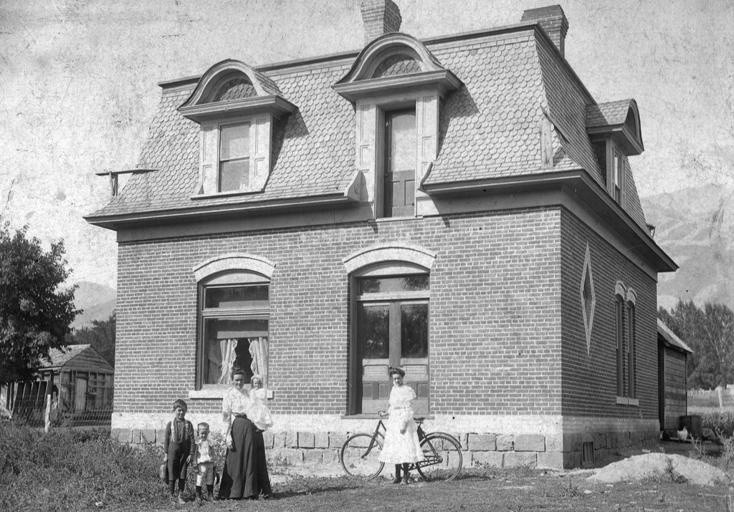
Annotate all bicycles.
[342,409,463,483]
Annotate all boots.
[392,463,408,485]
[167,479,185,505]
[195,485,203,506]
[206,483,217,505]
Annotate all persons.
[248,374,273,432]
[161,398,194,504]
[376,365,425,486]
[191,421,218,505]
[219,367,271,500]
